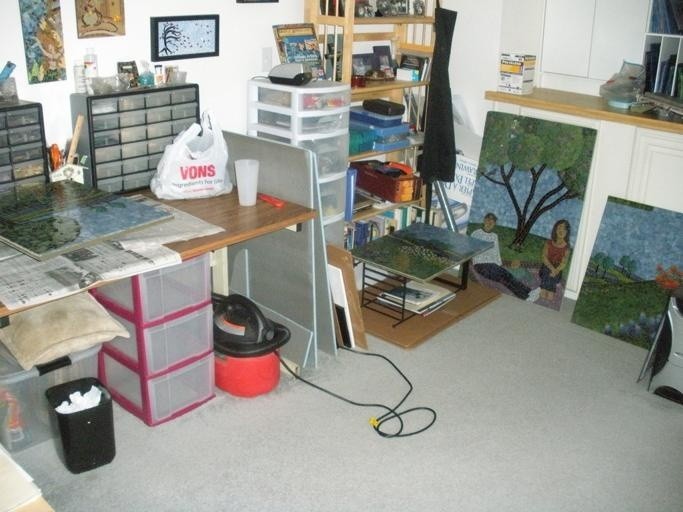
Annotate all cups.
[234,159,258,206]
[72,65,86,94]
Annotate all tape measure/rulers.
[67,115,84,164]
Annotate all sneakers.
[525,287,541,303]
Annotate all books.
[646,44,683,99]
[345,205,425,246]
[379,281,455,317]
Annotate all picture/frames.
[150,14,219,62]
[273,23,329,82]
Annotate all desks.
[345,223,494,328]
[0,178,318,324]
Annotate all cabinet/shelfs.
[640,0,682,110]
[303,0,436,225]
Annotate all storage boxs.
[246,74,351,250]
[69,82,200,196]
[0,341,103,454]
[87,253,218,427]
[0,99,48,197]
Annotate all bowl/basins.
[107,72,129,92]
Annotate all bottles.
[154,65,162,84]
[83,48,99,95]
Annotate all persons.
[470,213,542,303]
[539,221,571,301]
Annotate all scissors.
[50,144,59,172]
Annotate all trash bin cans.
[45,377,115,474]
